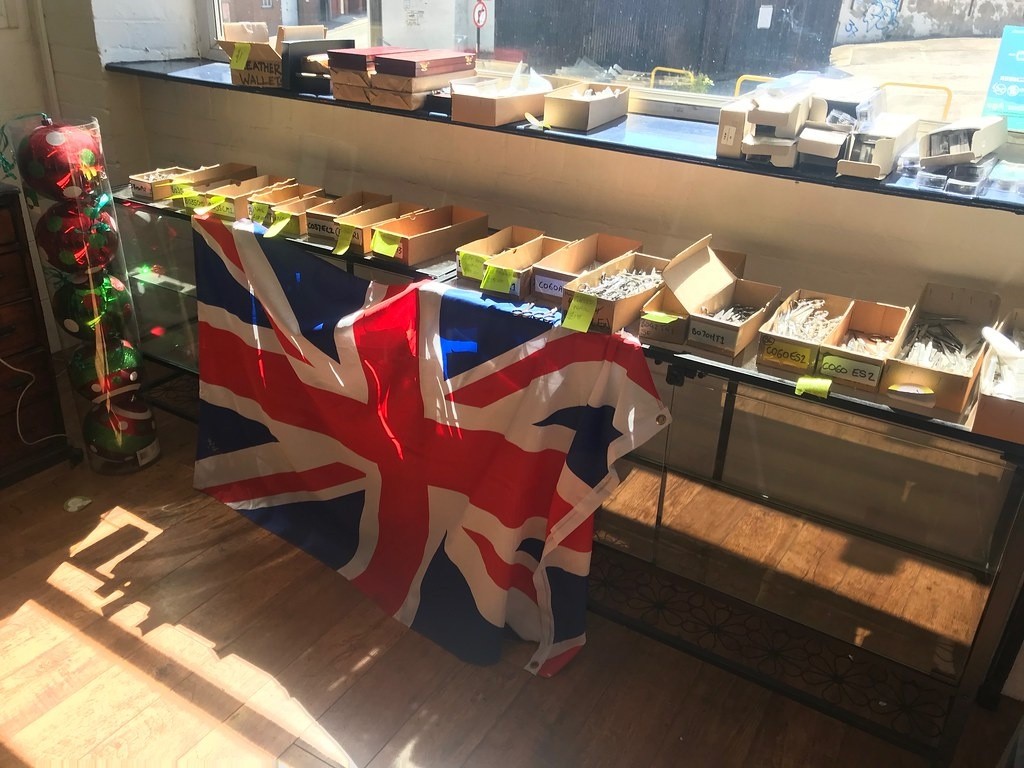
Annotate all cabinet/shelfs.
[111,187,1024,768]
[0,191,69,493]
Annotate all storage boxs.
[129,23,1024,443]
